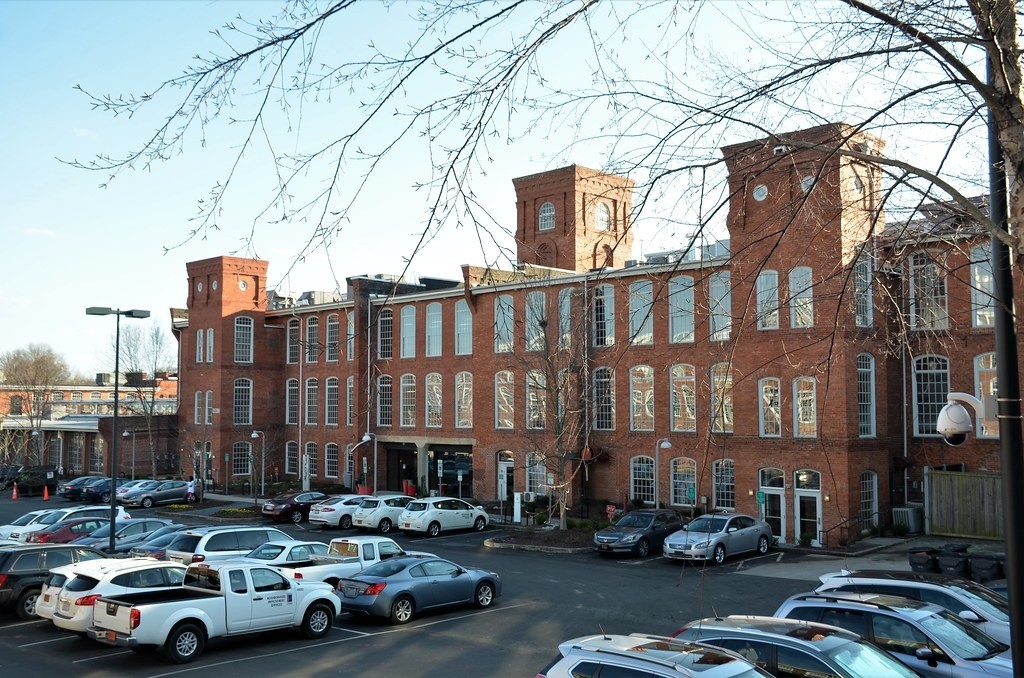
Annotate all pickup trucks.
[88,562,341,665]
[269,537,442,590]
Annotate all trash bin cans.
[970,556,1004,583]
[910,542,971,577]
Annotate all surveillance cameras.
[936,402,973,446]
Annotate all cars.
[58,475,204,509]
[339,556,503,626]
[352,494,418,533]
[70,519,184,559]
[35,555,158,639]
[262,491,332,523]
[398,497,490,539]
[308,495,373,529]
[662,513,773,563]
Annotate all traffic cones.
[11,482,19,499]
[42,486,49,500]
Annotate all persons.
[187,476,195,503]
[358,474,364,487]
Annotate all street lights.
[251,429,265,497]
[361,432,378,495]
[655,437,673,510]
[32,430,44,467]
[123,431,136,480]
[85,305,151,552]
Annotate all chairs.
[300,548,307,557]
[716,522,724,532]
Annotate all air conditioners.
[524,492,537,502]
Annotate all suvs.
[0,544,113,621]
[783,571,1014,678]
[165,526,294,567]
[593,509,690,558]
[536,633,759,678]
[673,614,924,678]
[0,505,132,549]
